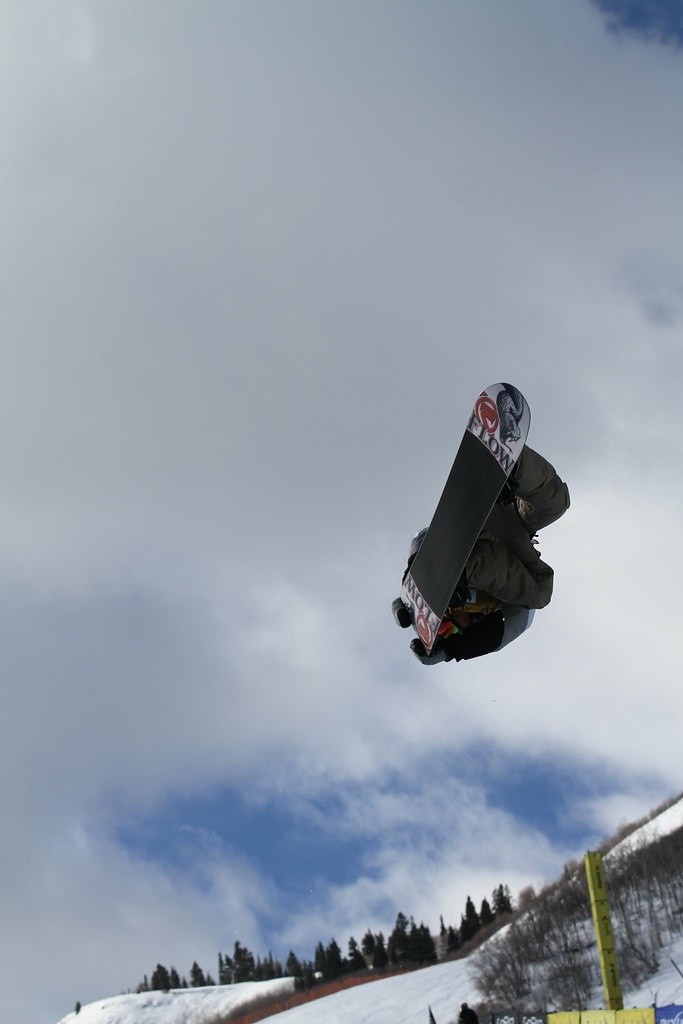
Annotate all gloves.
[410,639,446,665]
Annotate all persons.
[458,1003,480,1024]
[392,443,571,666]
[491,1012,496,1024]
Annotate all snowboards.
[401,382,531,658]
